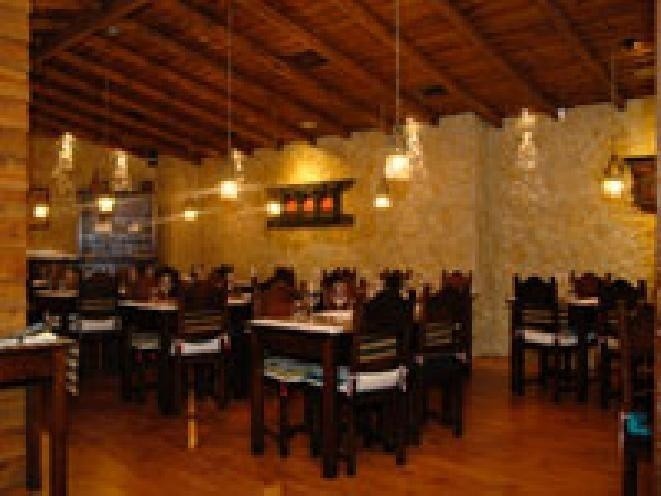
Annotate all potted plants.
[630,156,660,215]
[24,187,51,231]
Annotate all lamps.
[598,278,650,405]
[114,265,161,403]
[68,273,125,371]
[339,288,416,477]
[566,267,612,298]
[319,266,367,310]
[169,279,230,411]
[377,266,416,294]
[250,274,306,316]
[417,270,473,439]
[617,299,654,495]
[26,276,64,332]
[506,272,558,396]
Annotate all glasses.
[218,4,240,200]
[373,101,392,208]
[601,53,625,202]
[32,188,51,223]
[96,179,117,216]
[385,3,411,180]
[263,188,281,217]
[183,175,201,222]
